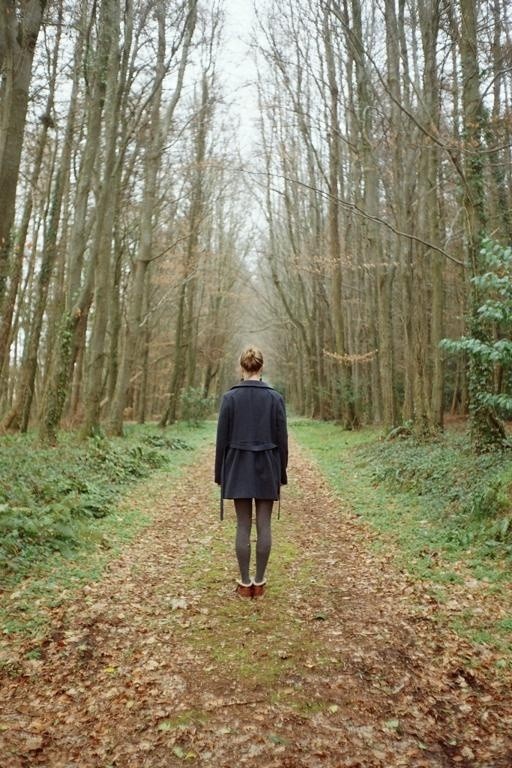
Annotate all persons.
[215,348,289,600]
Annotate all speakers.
[253,577,267,601]
[235,578,253,602]
